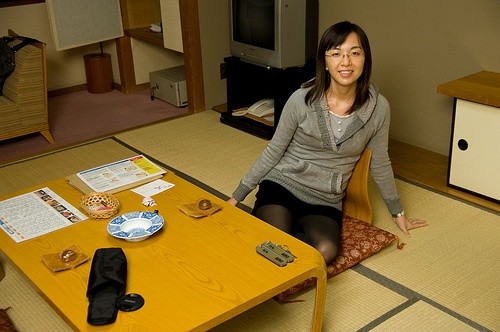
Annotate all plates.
[107,211,164,242]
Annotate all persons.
[226,20,430,264]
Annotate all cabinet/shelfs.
[119,0,185,54]
[219,57,312,142]
[436,69,499,205]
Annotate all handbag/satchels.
[0,36,34,96]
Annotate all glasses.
[324,50,365,58]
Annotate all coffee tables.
[0,155,328,332]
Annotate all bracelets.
[391,210,406,218]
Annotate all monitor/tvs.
[230,0,318,70]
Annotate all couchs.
[0,29,54,145]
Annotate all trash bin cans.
[83,53,114,94]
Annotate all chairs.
[344,149,373,224]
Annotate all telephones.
[248,99,274,117]
